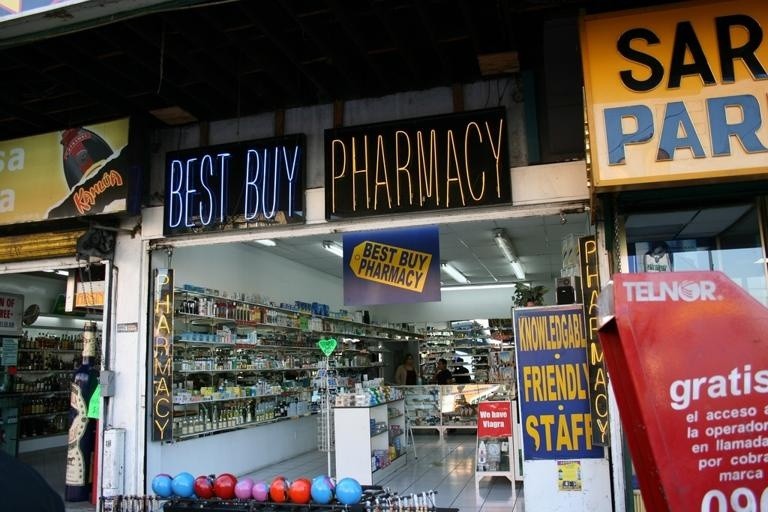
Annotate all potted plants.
[512,281,547,306]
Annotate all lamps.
[493,230,520,263]
[320,238,343,258]
[440,261,471,286]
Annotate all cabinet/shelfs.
[332,397,408,488]
[472,397,520,506]
[22,328,103,446]
[170,295,425,437]
[392,319,516,444]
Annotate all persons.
[1,447,65,512]
[451,358,471,383]
[395,354,418,385]
[433,359,451,383]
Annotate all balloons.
[152,473,173,498]
[171,472,195,497]
[310,476,334,504]
[288,477,312,504]
[194,475,213,499]
[214,474,236,499]
[270,476,290,503]
[233,478,254,499]
[334,478,363,506]
[251,480,269,502]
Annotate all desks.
[0,392,22,461]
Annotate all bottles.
[15,320,98,503]
[172,395,319,438]
[178,284,380,338]
[58,126,115,192]
[478,437,488,467]
